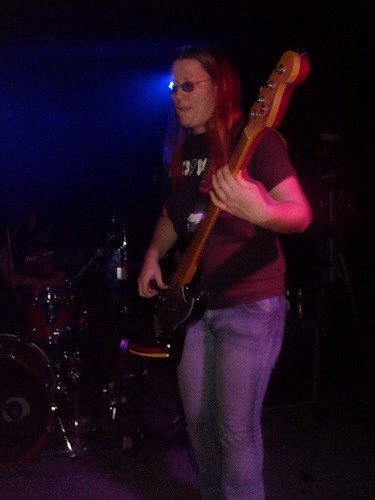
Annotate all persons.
[137,44,312,500]
[0,207,66,333]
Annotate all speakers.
[261,321,321,412]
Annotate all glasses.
[168,77,212,94]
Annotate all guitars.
[144,50,310,349]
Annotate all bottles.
[284,288,305,321]
[112,229,130,284]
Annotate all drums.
[13,270,76,340]
[0,332,56,468]
